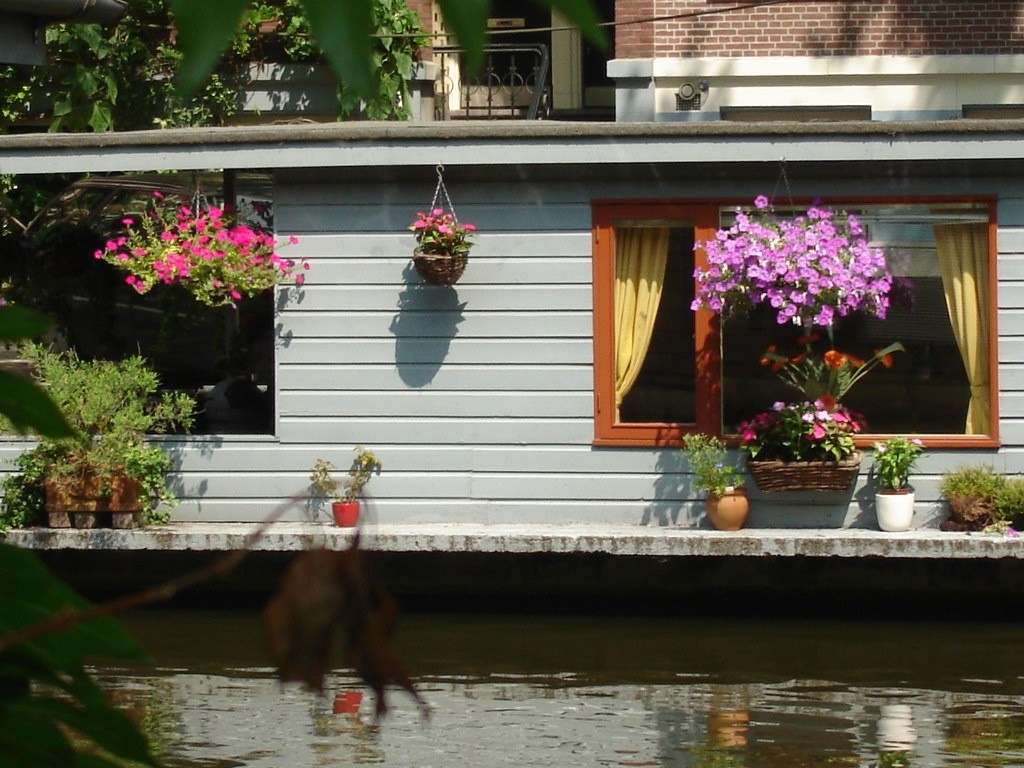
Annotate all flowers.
[92,180,312,312]
[736,393,866,462]
[686,190,894,330]
[406,207,477,253]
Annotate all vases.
[413,248,468,286]
[748,454,861,494]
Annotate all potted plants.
[687,709,749,768]
[16,337,204,530]
[865,435,928,532]
[309,444,381,529]
[680,433,749,531]
[873,703,916,768]
[309,689,385,766]
